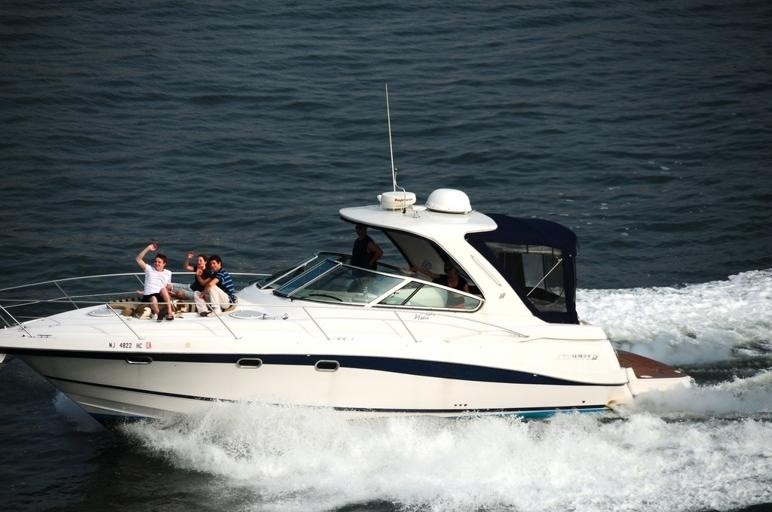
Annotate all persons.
[352,223,383,291]
[411,260,469,307]
[194,255,237,317]
[176,251,211,299]
[136,242,174,320]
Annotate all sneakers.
[157,312,174,321]
[199,310,222,317]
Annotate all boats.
[0,82,700,448]
